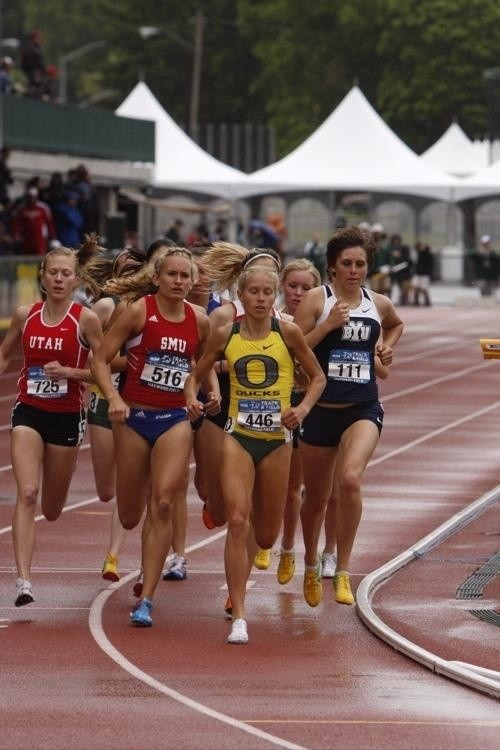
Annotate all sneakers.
[14,578,34,607]
[102,504,355,644]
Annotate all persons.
[0,147,104,213]
[1,215,413,646]
[413,241,436,307]
[0,24,58,106]
[467,235,500,299]
